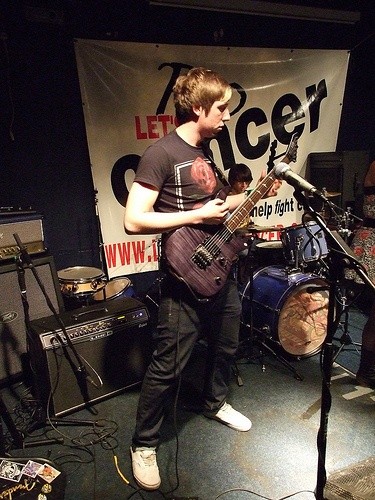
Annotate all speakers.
[27,295,154,418]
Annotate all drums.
[293,221,330,262]
[241,265,344,358]
[253,237,288,265]
[56,265,105,310]
[96,276,136,303]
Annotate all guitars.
[160,131,299,302]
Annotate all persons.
[123,67,282,490]
[225,163,267,256]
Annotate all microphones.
[77,368,94,386]
[275,162,329,202]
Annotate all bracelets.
[245,188,254,198]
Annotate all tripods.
[232,233,303,380]
[332,289,362,362]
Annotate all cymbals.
[233,221,286,232]
[309,188,342,199]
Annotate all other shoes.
[130,444,161,489]
[214,402,252,432]
